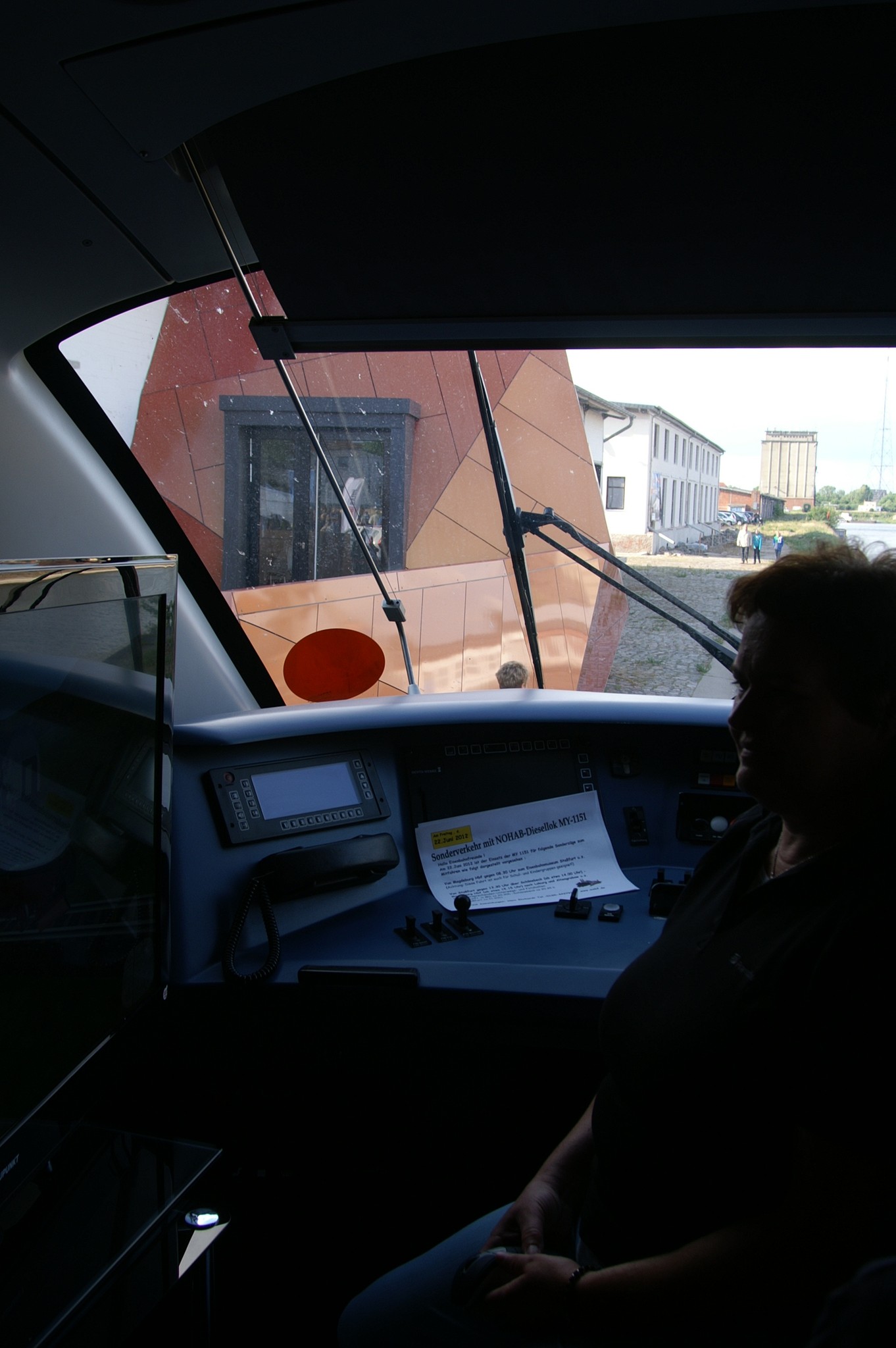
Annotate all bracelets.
[568,1265,594,1288]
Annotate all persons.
[736,524,752,564]
[773,531,784,561]
[342,540,896,1348]
[752,530,762,564]
[753,511,763,526]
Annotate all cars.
[718,510,759,526]
